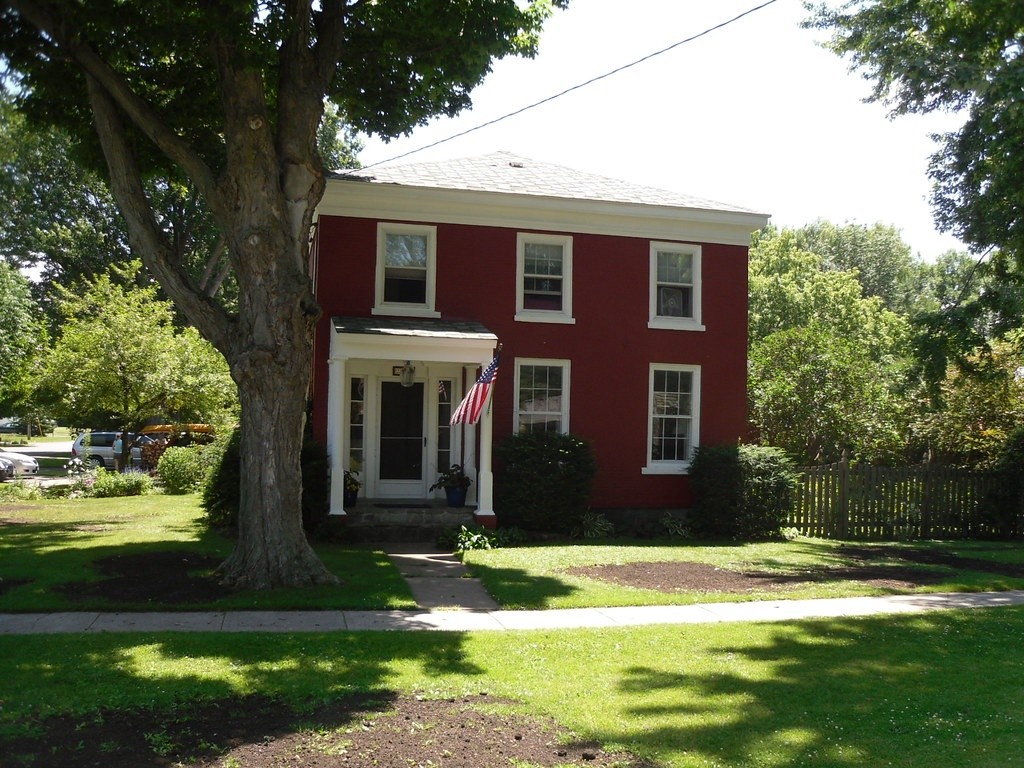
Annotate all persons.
[112,432,123,469]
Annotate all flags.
[450,355,499,426]
[439,382,445,394]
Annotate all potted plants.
[428,463,474,507]
[343,469,364,508]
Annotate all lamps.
[400,360,416,386]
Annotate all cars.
[0,448,39,483]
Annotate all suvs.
[71,424,214,471]
